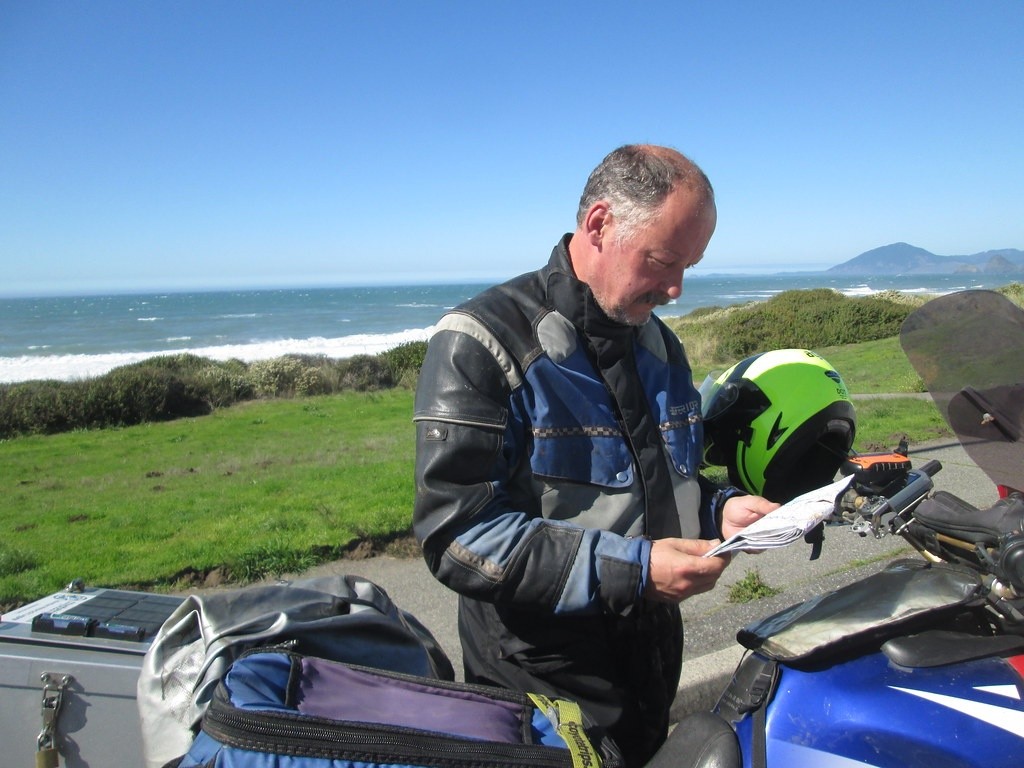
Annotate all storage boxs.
[1,585,202,768]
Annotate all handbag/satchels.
[177,646,622,768]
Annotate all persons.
[412,144,780,768]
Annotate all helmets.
[699,348,855,502]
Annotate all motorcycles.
[137,288,1024,768]
